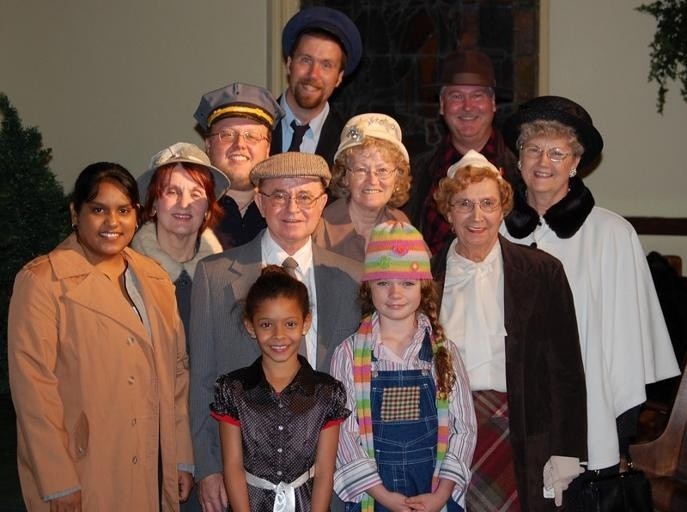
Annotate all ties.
[287,119,311,153]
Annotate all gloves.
[542,455,581,507]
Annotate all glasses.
[517,141,578,163]
[258,191,327,208]
[447,199,503,213]
[343,164,401,179]
[204,130,272,144]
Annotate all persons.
[7,162,196,512]
[328,219,478,512]
[269,6,363,205]
[402,49,525,255]
[208,265,352,512]
[432,148,585,511]
[192,82,286,250]
[313,112,412,263]
[127,141,233,358]
[189,152,363,512]
[502,96,682,489]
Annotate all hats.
[446,148,503,180]
[142,141,231,203]
[500,94,605,181]
[360,217,435,283]
[431,48,497,88]
[333,113,410,166]
[282,5,364,77]
[249,151,332,190]
[193,81,287,140]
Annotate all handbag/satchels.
[566,466,654,512]
